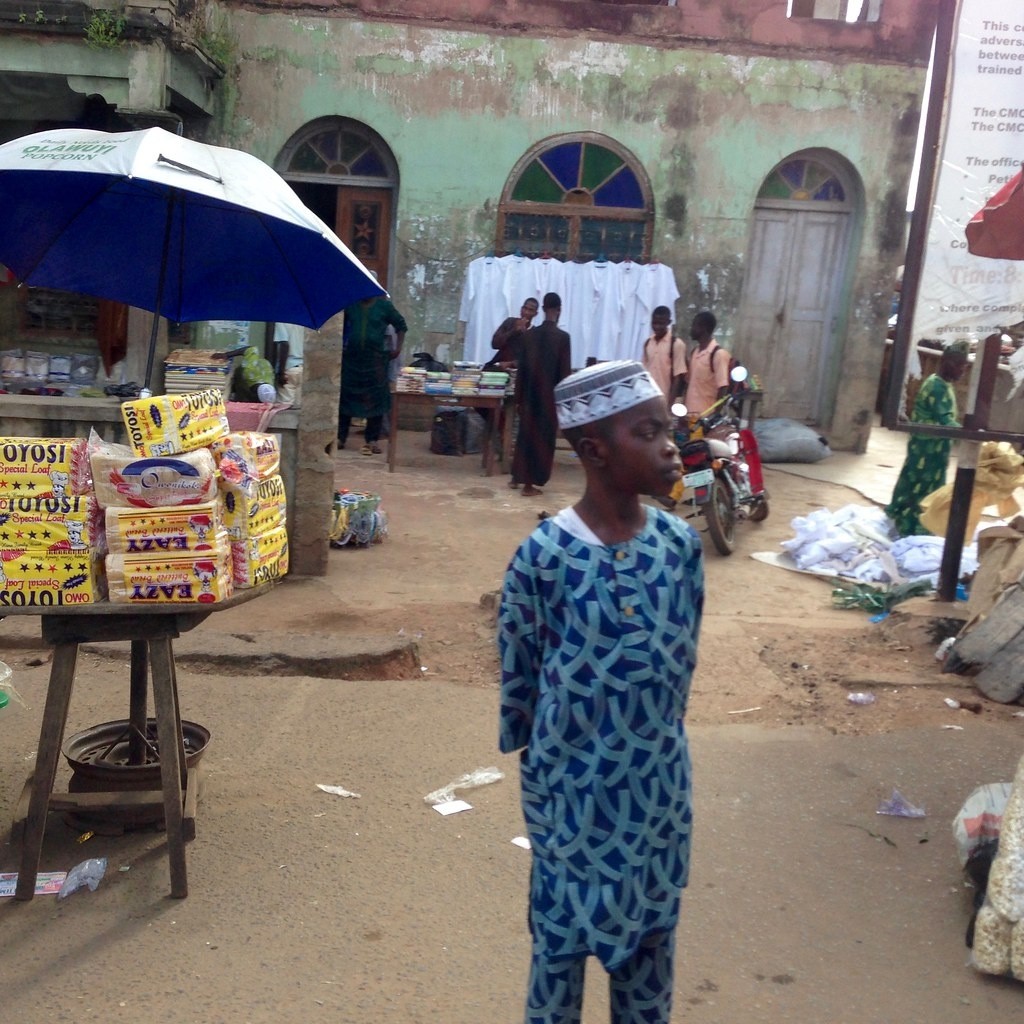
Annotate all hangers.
[624,253,630,271]
[648,252,659,270]
[572,253,582,262]
[595,251,608,268]
[514,247,522,257]
[539,248,550,259]
[484,245,494,257]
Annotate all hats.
[555,360,666,430]
[943,340,971,355]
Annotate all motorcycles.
[670,366,773,554]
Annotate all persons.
[886,339,971,538]
[509,292,574,497]
[502,351,709,1020]
[643,306,687,416]
[330,269,409,456]
[474,297,540,462]
[651,311,732,511]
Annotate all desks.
[1,579,277,904]
[735,384,765,429]
[388,381,515,477]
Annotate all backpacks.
[710,345,747,409]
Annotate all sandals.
[360,446,371,455]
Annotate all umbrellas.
[0,125,393,446]
[963,159,1024,264]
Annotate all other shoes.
[369,442,382,454]
